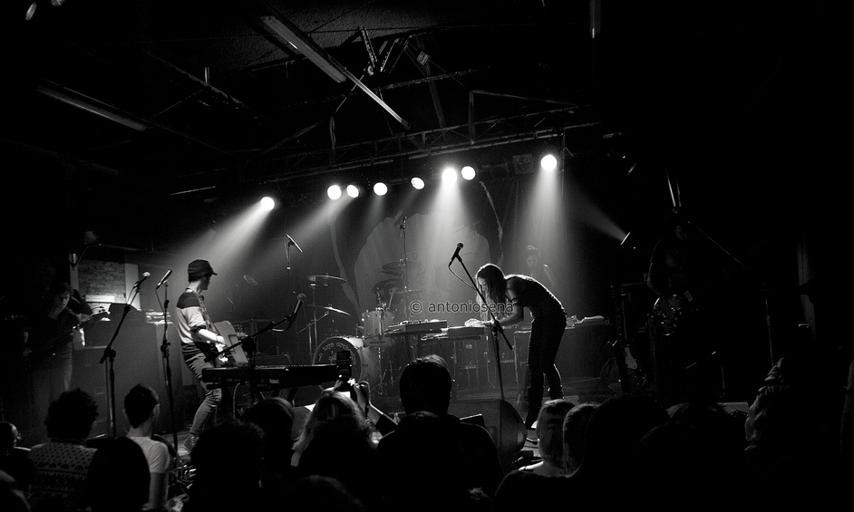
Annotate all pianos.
[201,364,340,383]
[383,319,448,340]
[426,325,488,341]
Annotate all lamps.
[259,190,281,212]
[461,160,479,182]
[346,182,364,198]
[326,182,343,201]
[538,143,560,172]
[411,171,427,191]
[373,180,389,196]
[440,160,459,181]
[618,231,640,250]
[16,2,37,20]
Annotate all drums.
[312,336,380,394]
[358,309,400,349]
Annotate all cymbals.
[321,304,349,319]
[309,274,345,288]
[393,287,421,301]
[381,259,417,278]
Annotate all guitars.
[602,288,646,391]
[202,322,232,367]
[52,307,110,352]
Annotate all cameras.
[333,348,367,403]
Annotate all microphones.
[133,270,151,288]
[282,231,304,256]
[155,266,174,290]
[284,292,307,331]
[448,242,464,267]
[400,215,408,231]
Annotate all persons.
[24,283,85,443]
[176,259,226,454]
[86,436,151,512]
[292,379,397,512]
[554,403,601,465]
[24,389,98,512]
[377,354,503,511]
[124,385,170,512]
[520,245,558,294]
[238,397,294,470]
[474,264,567,428]
[492,401,574,510]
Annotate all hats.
[187,260,218,282]
